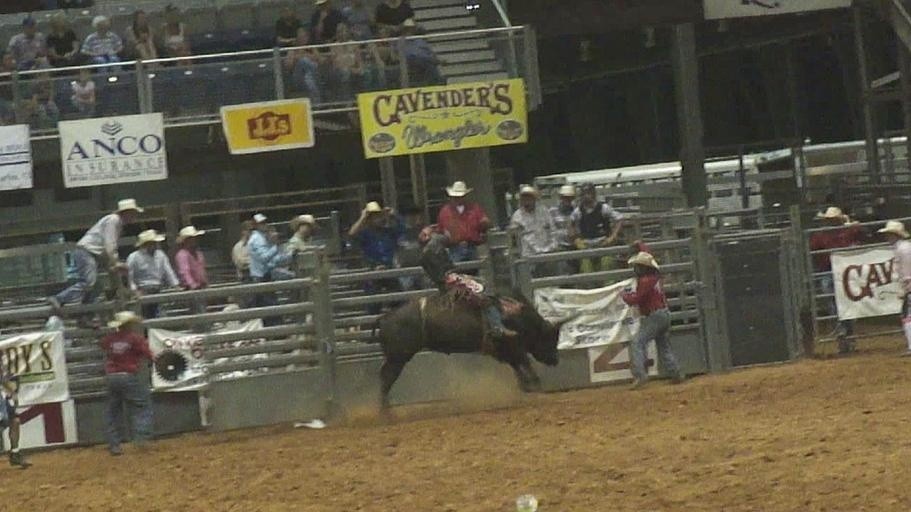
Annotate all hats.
[418,223,450,244]
[176,226,205,243]
[876,221,911,239]
[252,214,268,224]
[815,207,845,225]
[557,186,576,197]
[112,198,145,213]
[135,229,166,247]
[366,201,383,212]
[289,215,316,231]
[107,311,142,328]
[627,251,659,271]
[445,182,474,197]
[514,187,543,201]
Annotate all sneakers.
[9,452,33,467]
[629,374,648,390]
[493,326,518,336]
[672,374,685,383]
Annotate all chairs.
[0,0,439,130]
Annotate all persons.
[173,225,210,333]
[126,229,187,320]
[0,383,24,466]
[811,206,861,353]
[876,220,911,357]
[0,0,194,130]
[508,183,624,289]
[274,1,416,105]
[98,311,158,457]
[435,181,489,277]
[47,198,143,330]
[232,213,320,338]
[416,223,519,336]
[348,201,408,338]
[621,239,685,390]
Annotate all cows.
[371,284,585,421]
